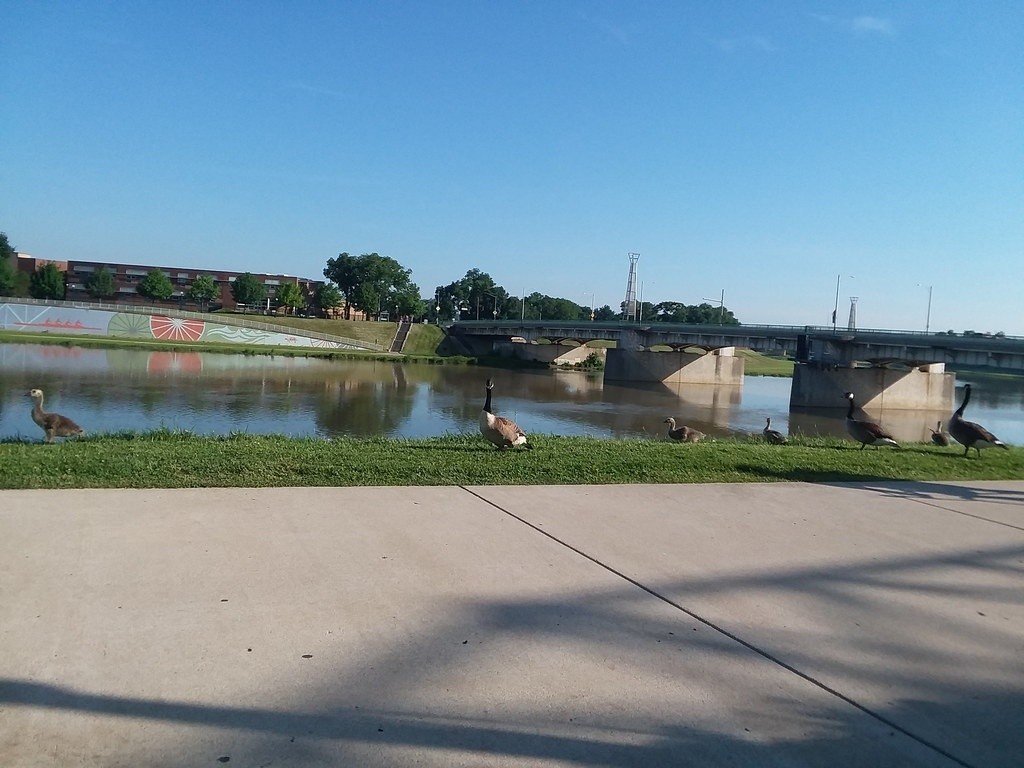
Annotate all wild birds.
[948,384,1010,457]
[928,421,950,447]
[664,417,707,443]
[840,392,902,450]
[479,379,533,452]
[762,418,788,445]
[24,389,84,442]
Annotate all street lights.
[352,302,357,321]
[71,284,75,301]
[275,297,279,317]
[179,291,183,309]
